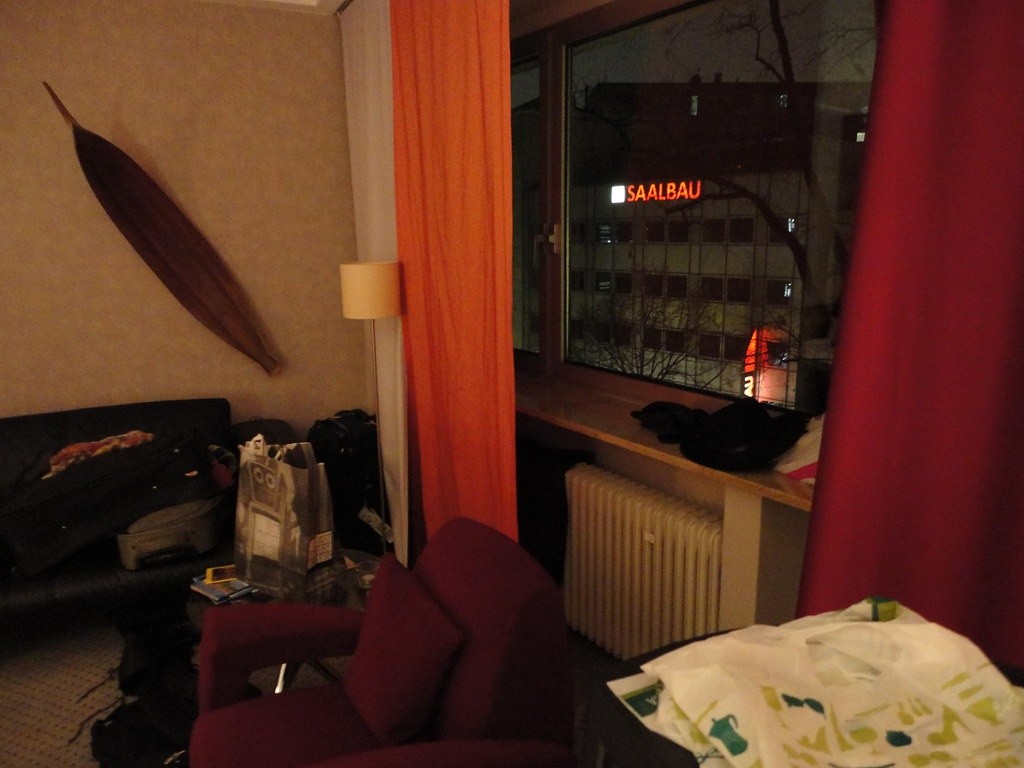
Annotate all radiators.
[562,461,723,662]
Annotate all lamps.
[340,260,402,555]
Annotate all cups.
[356,561,378,589]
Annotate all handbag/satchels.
[234,433,335,577]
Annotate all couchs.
[0,398,300,652]
[191,518,581,768]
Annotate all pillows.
[343,552,464,753]
